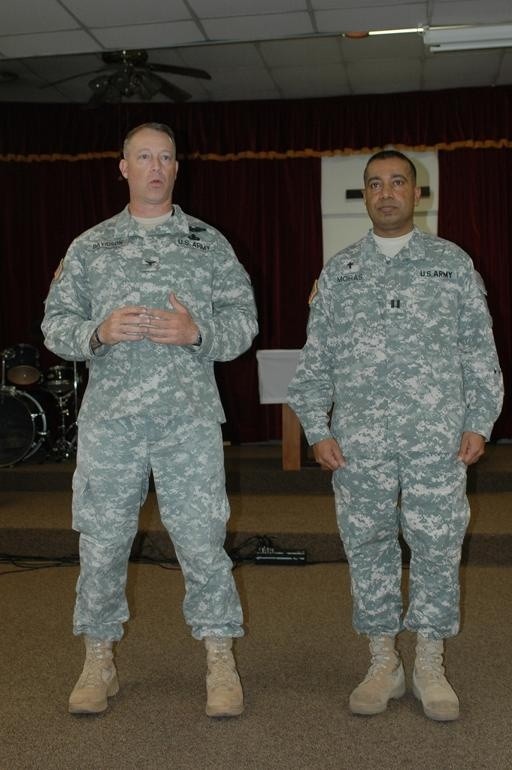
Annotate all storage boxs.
[256,348,305,404]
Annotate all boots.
[412,632,460,722]
[349,636,406,715]
[204,635,245,719]
[68,634,120,715]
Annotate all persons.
[41,123,261,716]
[287,149,504,722]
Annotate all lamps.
[89,49,152,102]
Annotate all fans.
[37,49,211,103]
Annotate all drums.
[0,344,79,468]
